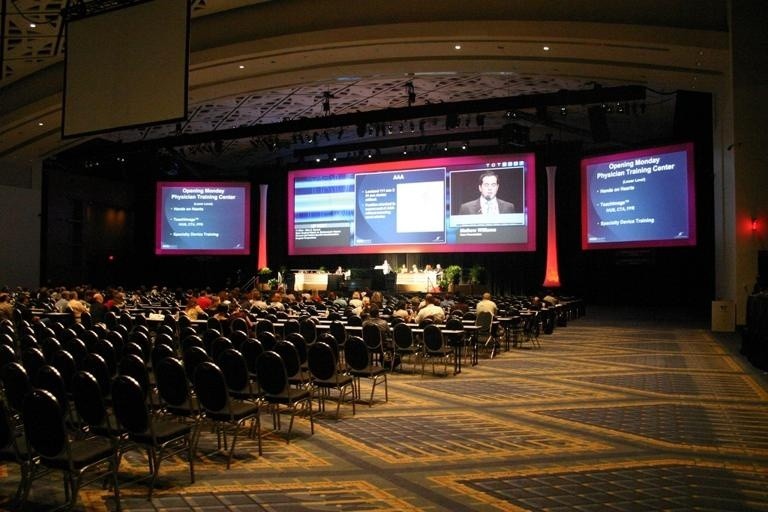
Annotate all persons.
[475,292,499,329]
[370,290,385,309]
[329,291,347,308]
[364,286,372,296]
[523,296,540,308]
[416,294,446,323]
[392,301,413,322]
[212,303,231,327]
[181,300,209,327]
[542,290,557,333]
[459,170,514,214]
[101,279,320,311]
[440,293,458,308]
[1,284,106,324]
[362,303,392,350]
[229,303,259,337]
[349,291,365,309]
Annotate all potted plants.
[437,278,448,291]
[269,278,278,290]
[257,267,271,284]
[469,265,486,296]
[445,265,461,294]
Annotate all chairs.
[215,349,277,439]
[390,323,423,377]
[286,334,312,389]
[111,375,196,500]
[1,363,28,433]
[29,366,76,429]
[153,360,215,454]
[276,341,319,420]
[183,288,317,347]
[316,333,356,402]
[53,350,75,396]
[183,346,213,407]
[22,389,122,512]
[445,321,467,370]
[115,357,167,421]
[71,372,148,469]
[489,295,587,352]
[346,337,389,407]
[22,348,46,379]
[329,322,352,365]
[420,326,452,378]
[1,345,17,368]
[74,354,118,416]
[469,311,499,360]
[0,397,41,507]
[0,285,180,359]
[253,350,314,444]
[362,294,483,320]
[309,343,356,422]
[239,340,266,372]
[194,363,263,470]
[361,323,392,371]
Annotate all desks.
[320,295,361,331]
